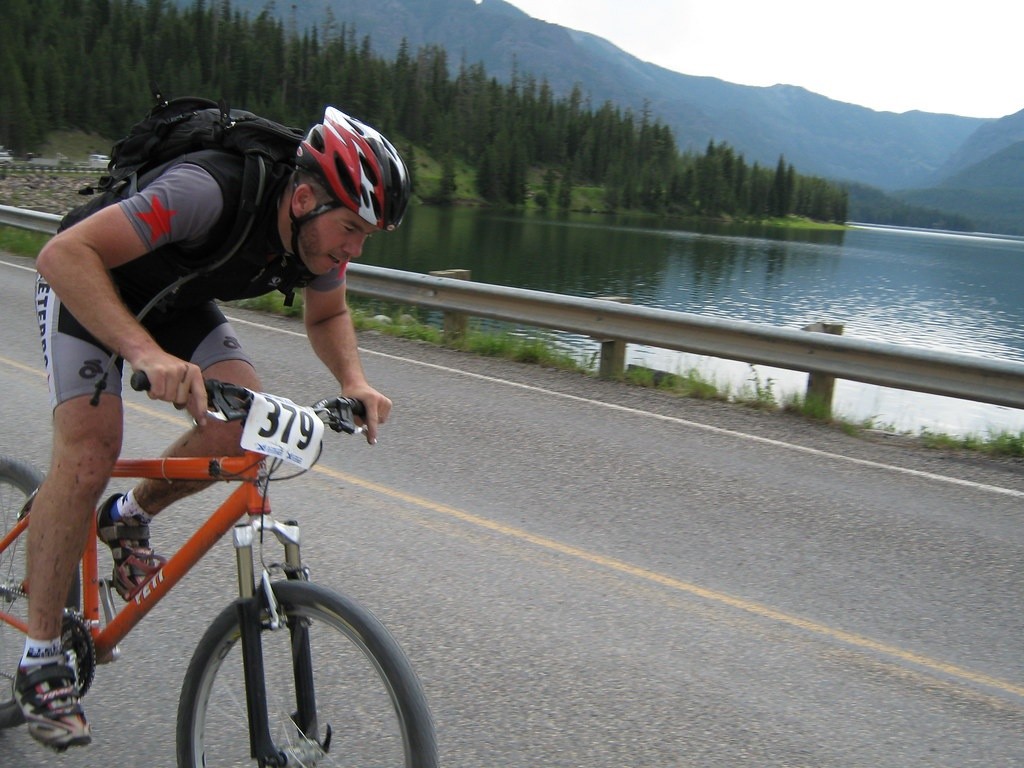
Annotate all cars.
[0,152,13,165]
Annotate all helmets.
[295,106,411,231]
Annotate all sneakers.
[96,492,166,601]
[12,654,92,749]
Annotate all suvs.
[88,154,110,164]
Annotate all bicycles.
[0,369,440,768]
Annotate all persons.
[11,105,409,750]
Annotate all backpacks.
[78,81,316,308]
